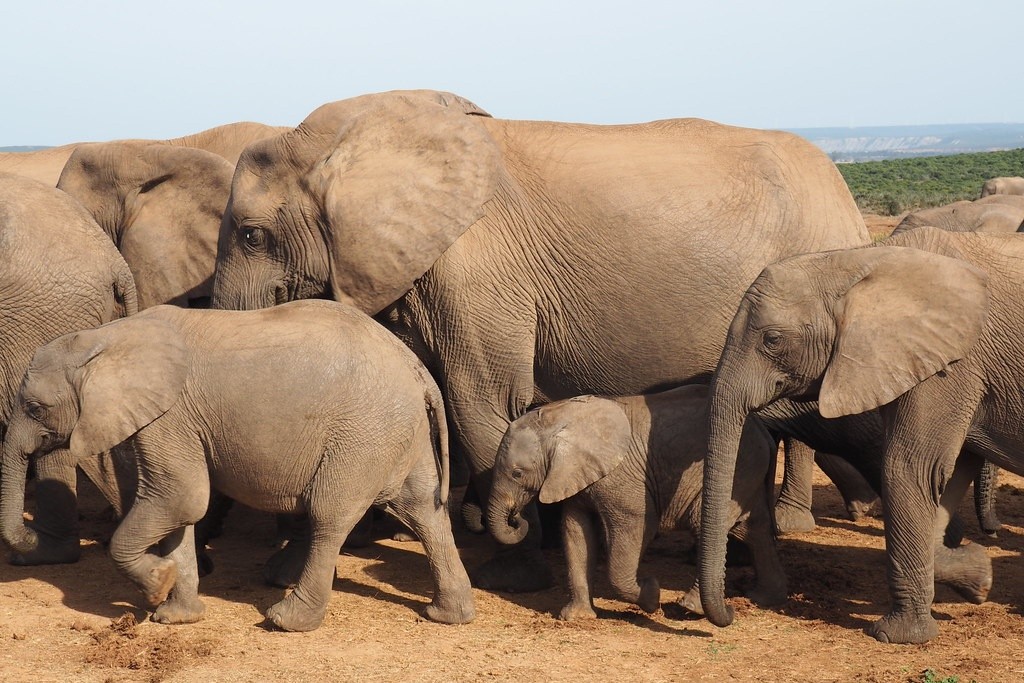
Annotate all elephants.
[1,89,1023,645]
